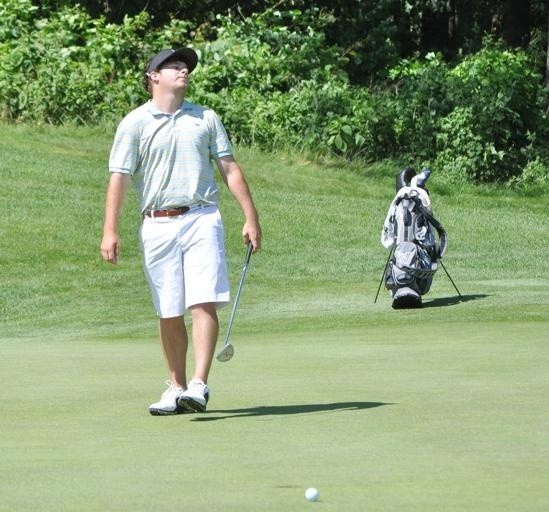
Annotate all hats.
[144,47,198,77]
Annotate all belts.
[145,202,209,219]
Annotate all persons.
[99,46,263,416]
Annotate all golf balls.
[305,488,319,500]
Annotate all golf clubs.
[216,241,252,362]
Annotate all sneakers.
[149,379,183,413]
[178,379,210,413]
